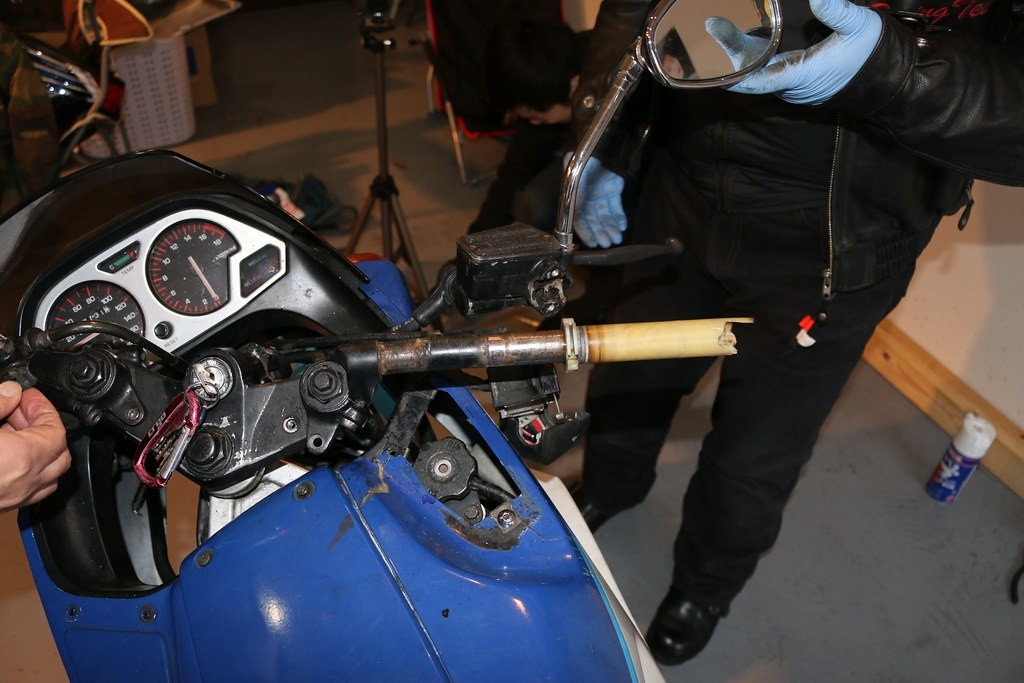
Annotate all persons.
[431,0,1024,666]
[0,379,72,510]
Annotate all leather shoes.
[569,478,625,533]
[646,583,721,665]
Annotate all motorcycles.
[0,2,782,683]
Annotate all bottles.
[11,54,60,200]
[925,412,997,503]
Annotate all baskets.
[78,36,196,157]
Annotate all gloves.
[719,0,882,106]
[565,152,629,249]
[705,16,770,71]
